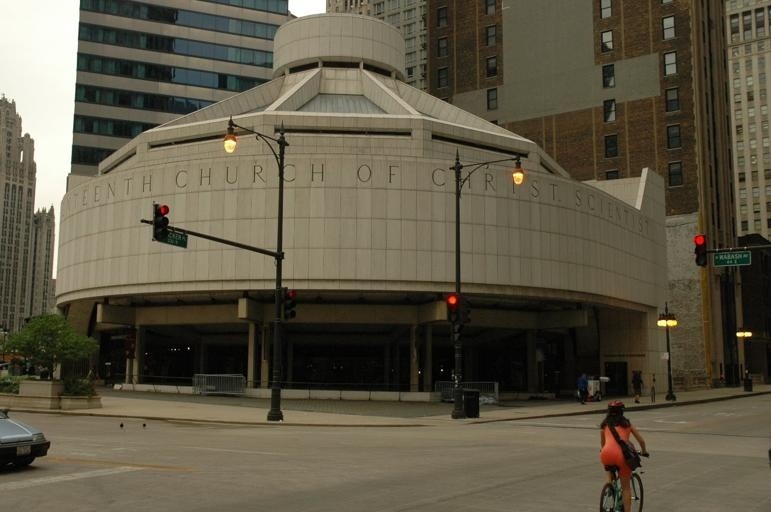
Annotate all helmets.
[607,400,625,408]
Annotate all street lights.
[656,302,680,401]
[0,329,9,361]
[449,147,531,422]
[222,114,286,422]
[733,326,755,394]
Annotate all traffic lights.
[443,291,462,329]
[150,203,171,243]
[691,235,709,271]
[280,285,297,324]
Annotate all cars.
[0,408,49,471]
[0,359,42,375]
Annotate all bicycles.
[590,448,651,512]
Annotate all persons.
[600,399,650,511]
[630,370,646,403]
[579,373,589,405]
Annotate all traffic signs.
[712,252,751,268]
[152,226,186,249]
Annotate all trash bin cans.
[463,389,480,419]
[744,379,753,392]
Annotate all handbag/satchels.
[623,446,641,471]
